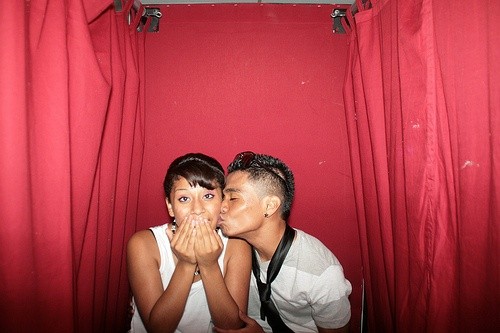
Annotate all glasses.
[227,150,290,195]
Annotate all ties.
[252,223,296,333]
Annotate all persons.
[126,153,252,333]
[217,151,353,333]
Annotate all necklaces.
[171,218,218,277]
